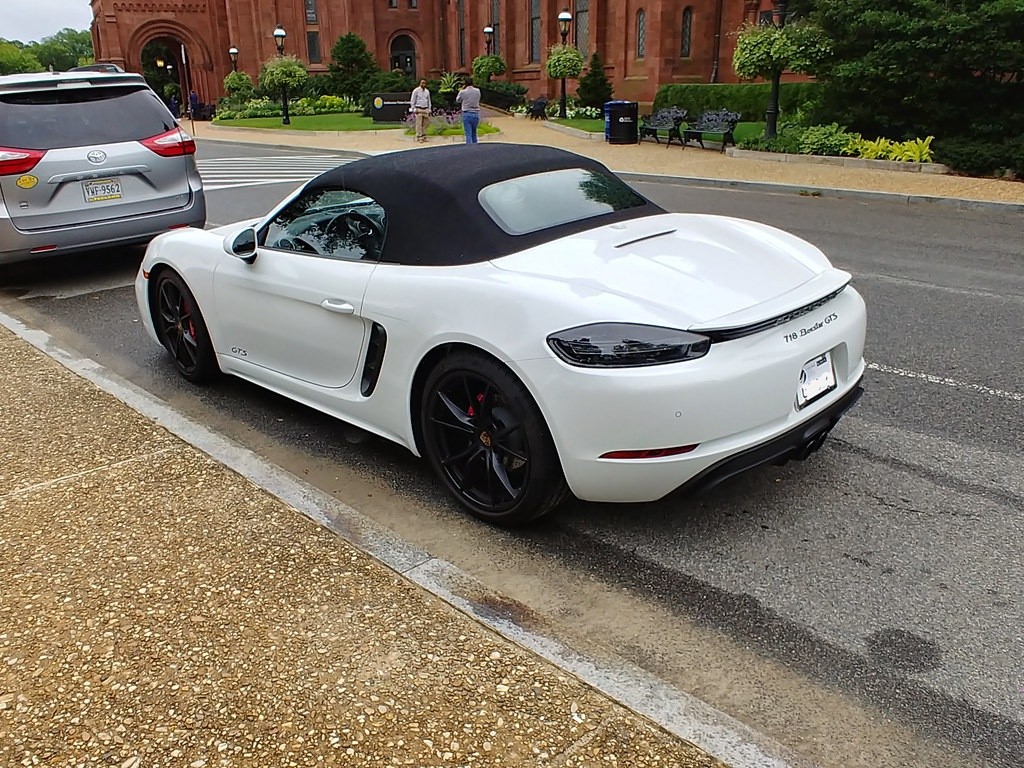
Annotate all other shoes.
[424,140,428,142]
[419,139,423,143]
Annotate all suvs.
[0,64,206,265]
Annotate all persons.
[170,95,179,118]
[411,79,431,143]
[187,96,191,120]
[456,78,480,143]
[190,90,198,120]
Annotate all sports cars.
[135,142,870,529]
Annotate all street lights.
[273,25,291,124]
[484,24,494,83]
[556,7,573,117]
[228,45,239,72]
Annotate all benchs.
[527,96,548,121]
[638,108,688,149]
[682,110,742,154]
[188,104,216,121]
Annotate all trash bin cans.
[190,102,205,120]
[604,99,638,145]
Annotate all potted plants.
[438,70,462,113]
